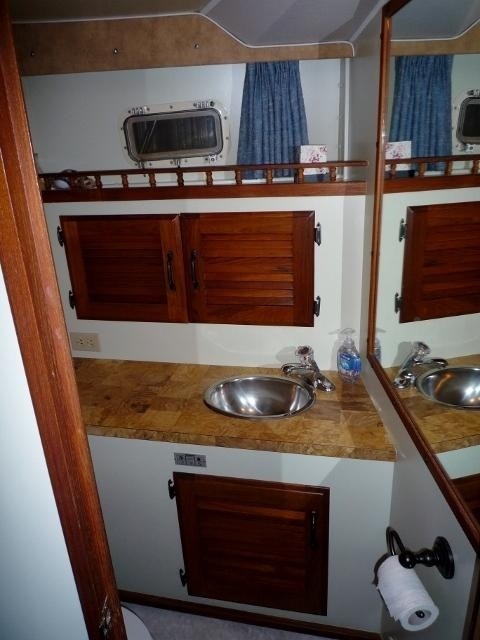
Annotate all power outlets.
[70,332,100,352]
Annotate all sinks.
[415,362,479,410]
[202,373,316,419]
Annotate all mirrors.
[367,0,480,556]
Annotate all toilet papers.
[374,554,440,631]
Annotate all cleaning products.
[336,326,361,383]
[373,326,387,362]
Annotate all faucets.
[392,342,450,390]
[281,346,335,392]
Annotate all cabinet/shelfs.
[168,473,330,616]
